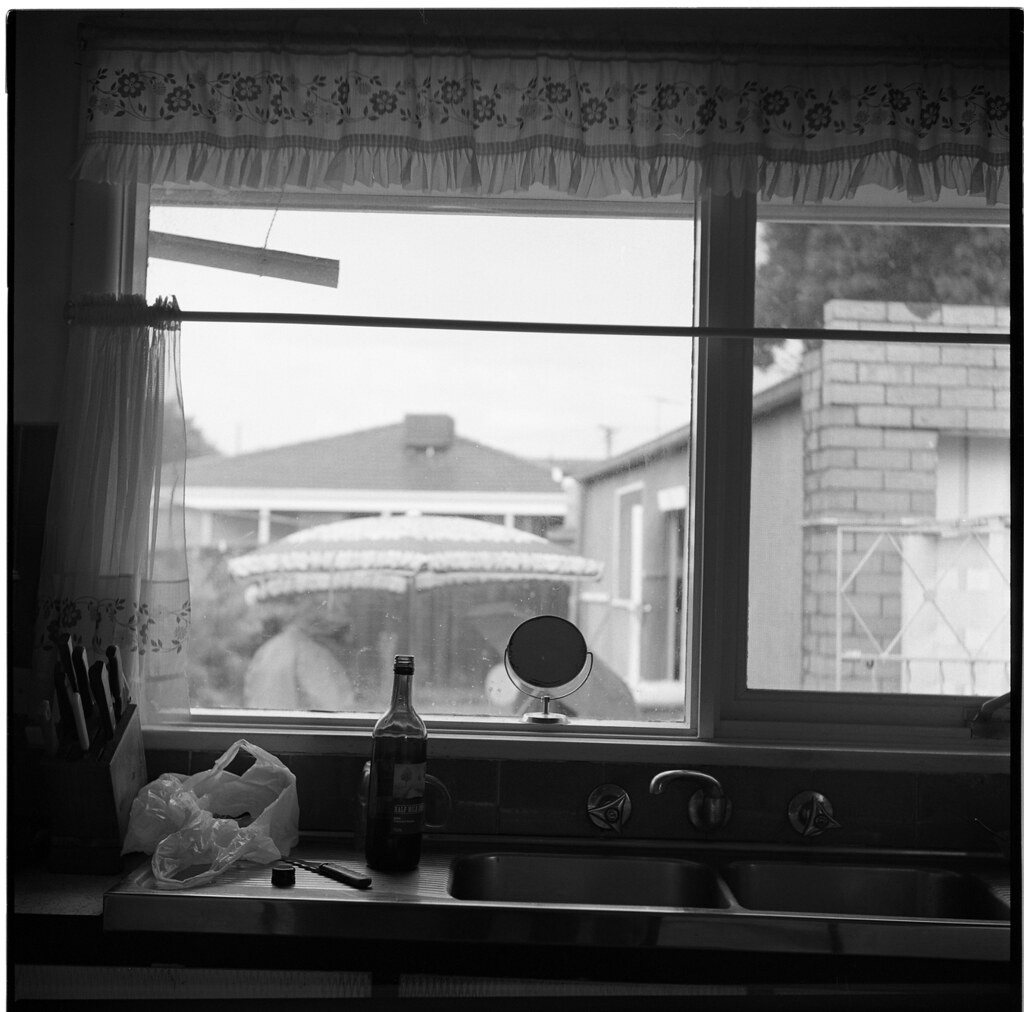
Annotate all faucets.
[649,769,732,830]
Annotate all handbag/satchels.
[120,739,299,888]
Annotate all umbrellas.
[467,598,640,721]
[223,511,602,653]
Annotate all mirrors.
[499,612,595,732]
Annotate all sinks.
[446,849,734,915]
[719,854,1017,927]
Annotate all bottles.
[365,654,427,870]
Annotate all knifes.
[278,858,373,888]
[54,634,130,761]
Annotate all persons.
[240,592,373,712]
